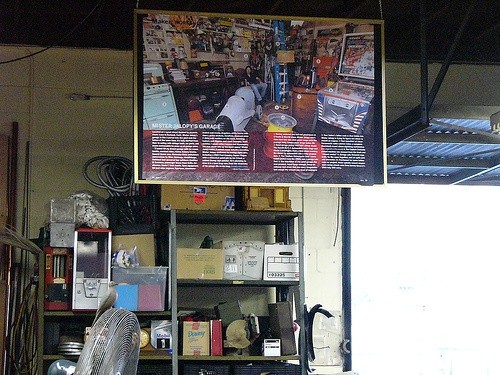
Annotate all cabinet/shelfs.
[34,208,308,375]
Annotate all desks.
[174,78,230,121]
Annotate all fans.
[48,307,140,375]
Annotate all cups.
[43,321,59,355]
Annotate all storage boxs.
[111,266,169,311]
[317,81,374,134]
[209,319,222,356]
[112,235,159,267]
[150,322,172,351]
[267,301,297,355]
[262,243,299,281]
[145,183,235,211]
[240,186,292,211]
[176,247,223,280]
[178,316,210,357]
[213,240,264,280]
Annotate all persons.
[240,65,268,105]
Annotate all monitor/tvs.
[133,7,387,188]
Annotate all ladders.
[273,21,290,104]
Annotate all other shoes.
[259,101,265,107]
[262,97,266,101]
